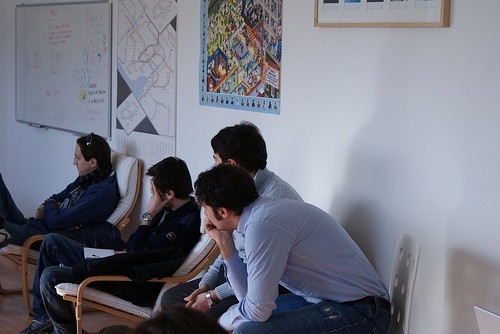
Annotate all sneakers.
[21,316,53,334]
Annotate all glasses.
[86,132,98,152]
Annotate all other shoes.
[0,228,13,248]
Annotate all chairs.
[54,208,233,334]
[0,152,143,314]
[390,232,421,334]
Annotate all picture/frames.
[314,0,452,29]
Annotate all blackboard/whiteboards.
[14,2,111,139]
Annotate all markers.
[30,124,42,127]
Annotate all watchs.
[205,291,216,308]
[141,212,152,222]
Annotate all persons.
[20,156,200,334]
[99,122,308,334]
[0,132,119,251]
[194,164,392,334]
